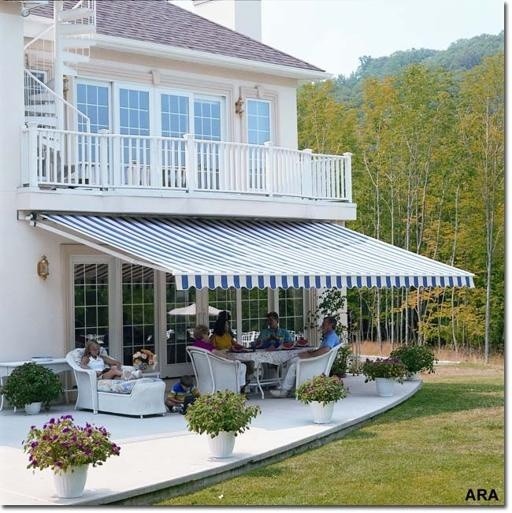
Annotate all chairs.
[65,346,168,420]
[185,343,247,404]
[293,338,348,401]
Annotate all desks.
[225,346,316,402]
[0,358,77,413]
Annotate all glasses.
[204,332,209,336]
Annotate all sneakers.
[171,405,179,412]
[270,390,288,397]
[123,370,134,381]
[131,370,142,378]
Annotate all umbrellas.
[168,301,230,315]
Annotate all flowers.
[131,349,158,370]
[20,416,120,472]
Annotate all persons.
[103,311,147,365]
[80,338,144,380]
[211,318,256,395]
[75,319,89,347]
[193,325,218,349]
[269,315,342,399]
[169,318,195,340]
[256,311,291,390]
[165,374,201,413]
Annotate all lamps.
[37,253,50,280]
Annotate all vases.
[49,462,88,497]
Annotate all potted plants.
[185,388,261,458]
[0,363,65,415]
[300,373,348,424]
[362,339,437,397]
